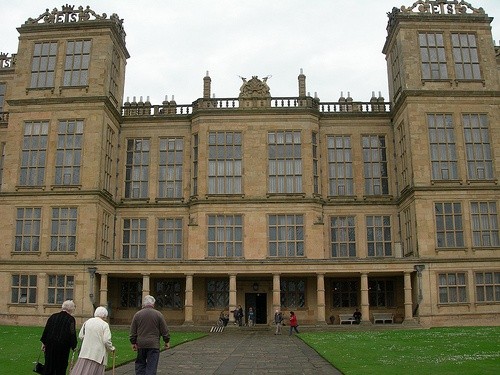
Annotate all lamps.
[253,280,259,291]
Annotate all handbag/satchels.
[32,350,45,374]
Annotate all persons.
[40,300,78,375]
[129,295,170,375]
[70,307,115,375]
[230,305,243,327]
[275,312,282,335]
[247,307,256,327]
[353,308,362,325]
[289,311,298,335]
[221,310,229,326]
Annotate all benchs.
[372,312,394,324]
[338,313,356,325]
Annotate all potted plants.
[330,314,335,324]
